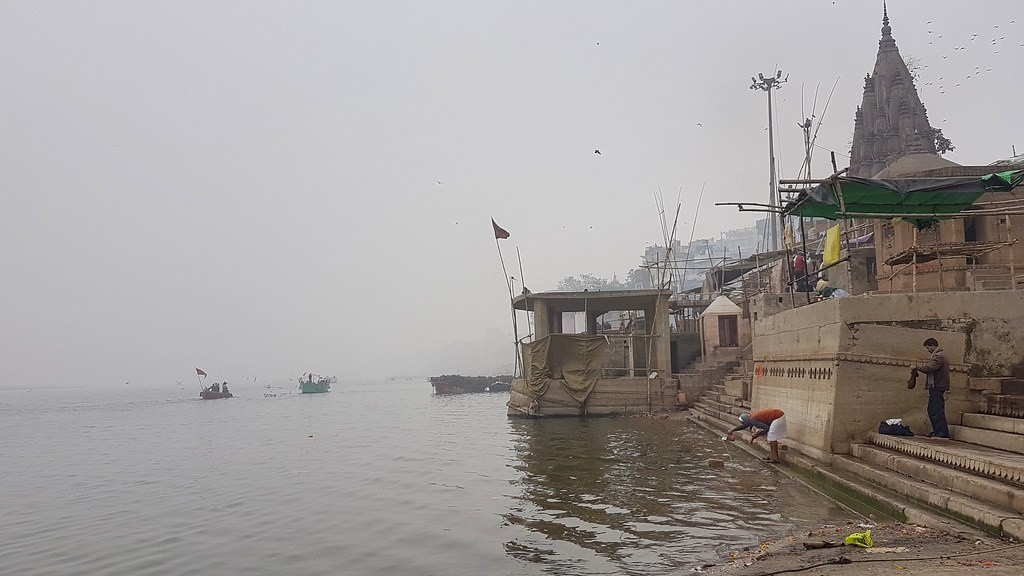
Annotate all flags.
[196,367,207,379]
[491,216,511,239]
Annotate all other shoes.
[766,456,780,463]
[928,432,950,440]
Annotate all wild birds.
[594,149,601,155]
[437,180,443,184]
[695,121,703,127]
[942,119,947,123]
[910,19,1024,95]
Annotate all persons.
[793,251,806,292]
[309,373,312,382]
[207,381,230,394]
[619,321,632,329]
[670,323,676,332]
[727,408,787,464]
[913,338,950,442]
[596,321,611,330]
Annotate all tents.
[778,167,1024,307]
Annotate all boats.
[301,382,331,393]
[199,392,234,400]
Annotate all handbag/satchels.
[879,421,914,436]
